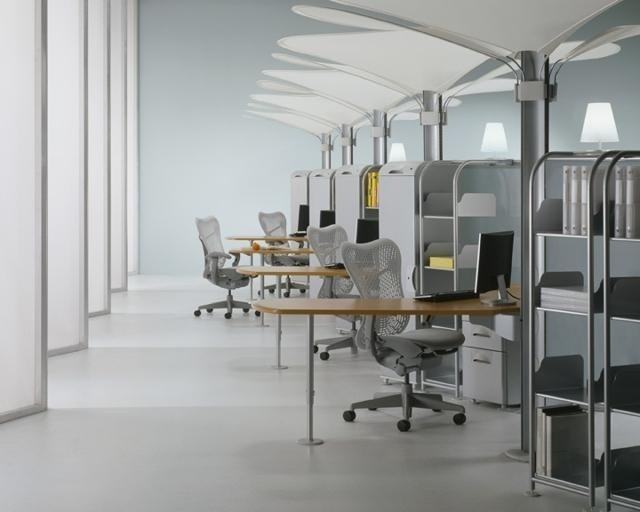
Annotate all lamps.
[581,101,622,152]
[479,121,509,161]
[387,143,407,162]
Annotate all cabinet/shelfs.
[526,149,619,512]
[418,162,495,391]
[460,317,524,407]
[603,146,640,511]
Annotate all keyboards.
[288,233,307,237]
[324,263,345,270]
[413,290,480,302]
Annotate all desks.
[236,262,360,325]
[249,282,524,447]
[222,210,326,329]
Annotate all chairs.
[339,238,469,430]
[191,215,263,319]
[307,224,382,359]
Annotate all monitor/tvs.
[474,231,518,307]
[356,218,379,243]
[319,209,335,228]
[298,204,309,231]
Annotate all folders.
[562,165,613,236]
[614,165,639,239]
